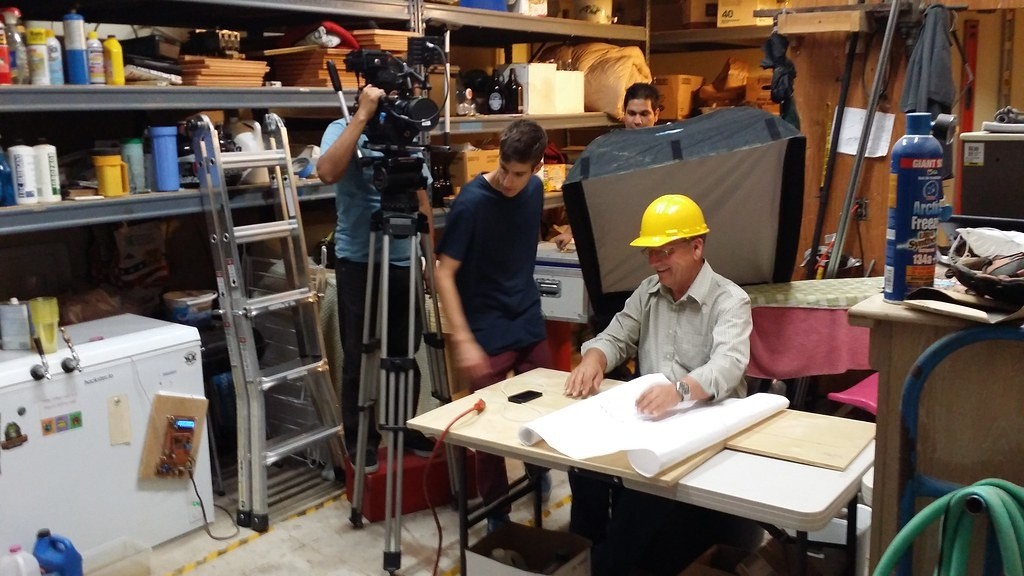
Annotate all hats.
[629,194,709,247]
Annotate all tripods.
[326,59,465,576]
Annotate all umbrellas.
[761,9,801,129]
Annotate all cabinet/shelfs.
[418,0,652,271]
[0,0,419,237]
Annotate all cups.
[93,155,129,197]
[455,89,471,116]
[149,126,180,192]
[29,295,61,353]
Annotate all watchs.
[675,380,691,401]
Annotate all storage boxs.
[426,71,464,117]
[681,0,779,32]
[531,241,596,325]
[677,542,755,576]
[650,56,781,126]
[446,144,588,193]
[494,62,585,115]
[138,203,343,468]
[463,522,590,576]
[960,130,1024,233]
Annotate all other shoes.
[526,465,554,495]
[489,517,511,534]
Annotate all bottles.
[883,112,943,305]
[504,68,523,114]
[485,68,505,113]
[120,137,145,193]
[0,7,125,87]
[0,135,63,207]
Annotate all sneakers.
[395,434,439,458]
[350,446,380,473]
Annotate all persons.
[564,194,753,576]
[555,83,663,248]
[433,119,546,532]
[317,84,440,475]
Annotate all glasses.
[641,248,676,258]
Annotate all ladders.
[186,110,350,534]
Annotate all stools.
[827,371,878,421]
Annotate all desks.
[846,266,1024,576]
[738,275,886,398]
[406,366,875,576]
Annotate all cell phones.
[508,390,542,404]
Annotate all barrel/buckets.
[0,545,40,576]
[162,289,218,319]
[228,117,269,183]
[32,528,83,576]
